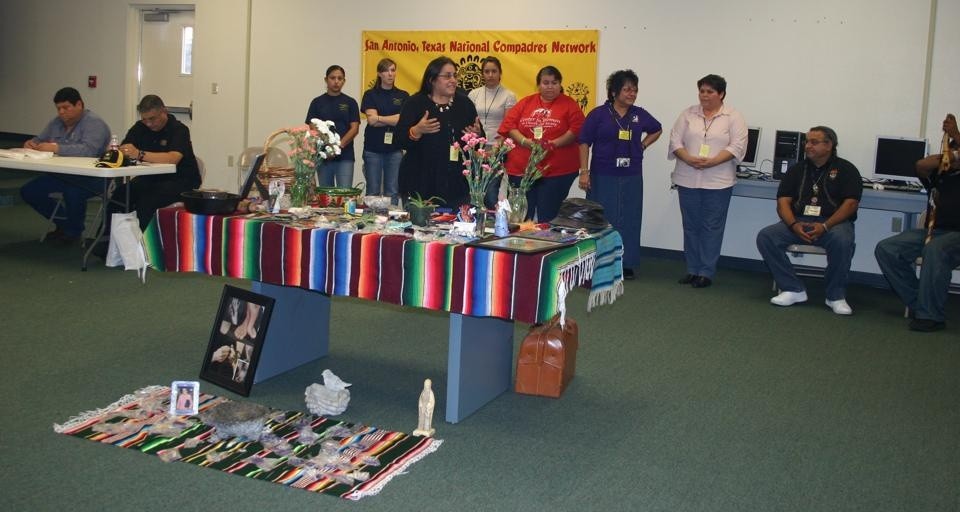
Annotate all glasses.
[140,110,160,122]
[805,139,829,145]
[437,73,458,79]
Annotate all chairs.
[904,210,960,318]
[195,157,205,179]
[238,147,287,192]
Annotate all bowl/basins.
[179,190,241,216]
[316,186,362,207]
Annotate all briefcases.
[514,311,579,399]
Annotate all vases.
[507,188,528,223]
[470,193,487,238]
[290,179,307,208]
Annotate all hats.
[549,197,609,231]
[95,150,130,169]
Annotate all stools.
[38,177,116,248]
[773,244,826,295]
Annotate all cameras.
[617,158,631,169]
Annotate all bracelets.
[789,220,801,228]
[822,223,830,233]
[520,137,527,146]
[641,142,648,150]
[409,129,416,139]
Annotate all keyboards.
[736,171,751,180]
[863,178,923,192]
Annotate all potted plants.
[405,192,447,226]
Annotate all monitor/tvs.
[872,135,929,181]
[736,127,764,171]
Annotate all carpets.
[54,386,443,499]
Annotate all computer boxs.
[773,130,806,180]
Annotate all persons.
[86,95,202,269]
[305,65,361,188]
[20,88,111,242]
[497,66,587,224]
[756,126,863,315]
[577,69,662,279]
[176,387,194,409]
[212,334,233,382]
[360,59,411,196]
[667,76,747,289]
[467,56,517,212]
[397,57,486,208]
[874,113,960,332]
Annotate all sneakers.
[824,297,853,316]
[770,289,809,307]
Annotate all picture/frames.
[239,154,269,200]
[465,235,567,254]
[200,283,276,396]
[172,381,200,416]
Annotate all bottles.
[110,134,118,151]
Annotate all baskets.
[255,128,304,209]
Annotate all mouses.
[873,183,884,191]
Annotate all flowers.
[454,132,515,193]
[483,139,557,192]
[286,118,341,178]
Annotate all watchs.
[138,149,144,161]
[578,168,591,175]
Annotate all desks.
[0,151,176,271]
[732,176,928,230]
[142,204,623,423]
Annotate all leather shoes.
[623,267,634,281]
[678,273,697,284]
[692,276,712,288]
[908,317,946,333]
